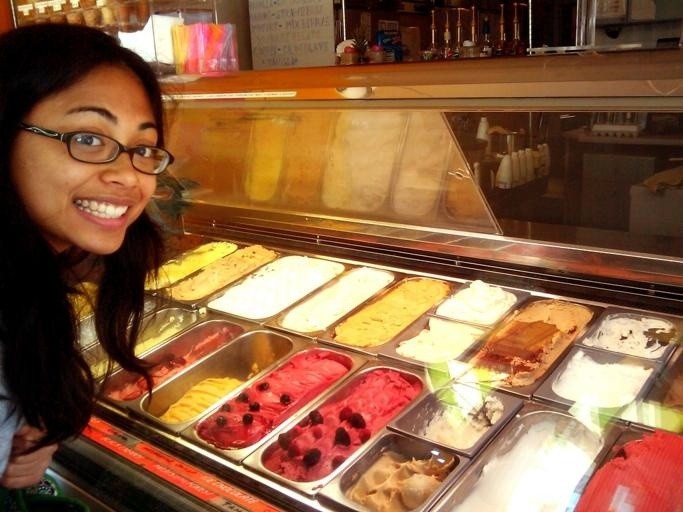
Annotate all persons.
[0,22,180,512]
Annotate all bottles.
[477,116,491,157]
[368,46,385,64]
[494,145,551,189]
[345,47,358,64]
[423,41,525,60]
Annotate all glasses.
[23,123,175,178]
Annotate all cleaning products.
[425,2,527,57]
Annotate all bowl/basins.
[495,129,524,154]
